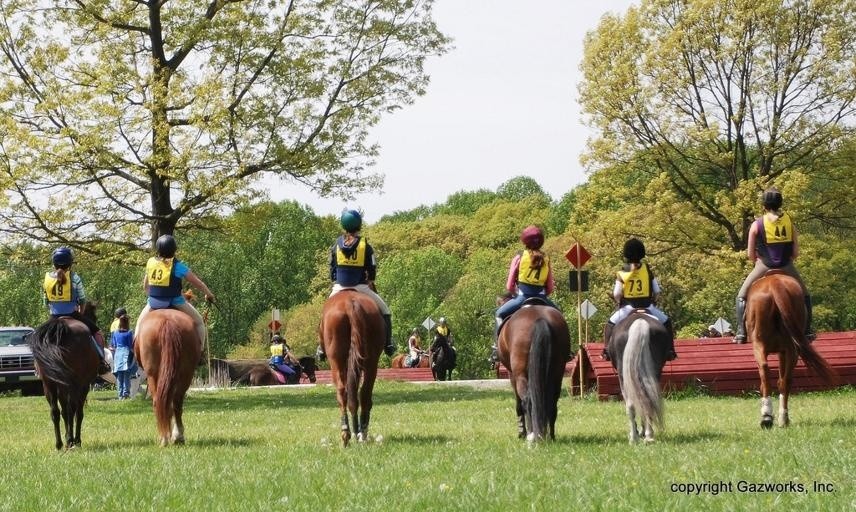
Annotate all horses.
[133,288,204,445]
[318,289,388,448]
[744,273,834,430]
[607,311,672,445]
[240,357,317,386]
[496,294,571,442]
[430,328,457,381]
[392,346,432,368]
[29,301,102,451]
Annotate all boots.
[317,345,325,362]
[663,319,675,360]
[383,314,397,357]
[804,295,815,341]
[98,345,112,375]
[601,323,613,361]
[488,313,505,362]
[734,296,748,343]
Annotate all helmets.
[342,211,361,232]
[156,234,177,256]
[115,308,126,319]
[271,335,279,343]
[439,317,446,324]
[412,328,419,333]
[761,187,782,208]
[521,226,543,247]
[624,238,646,260]
[53,248,72,267]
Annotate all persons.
[110,308,127,332]
[111,316,134,400]
[315,210,399,361]
[42,247,112,371]
[435,318,451,347]
[487,225,575,362]
[283,339,300,365]
[132,234,217,366]
[270,336,296,384]
[598,236,679,361]
[733,191,819,343]
[409,328,424,367]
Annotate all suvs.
[0,327,37,376]
